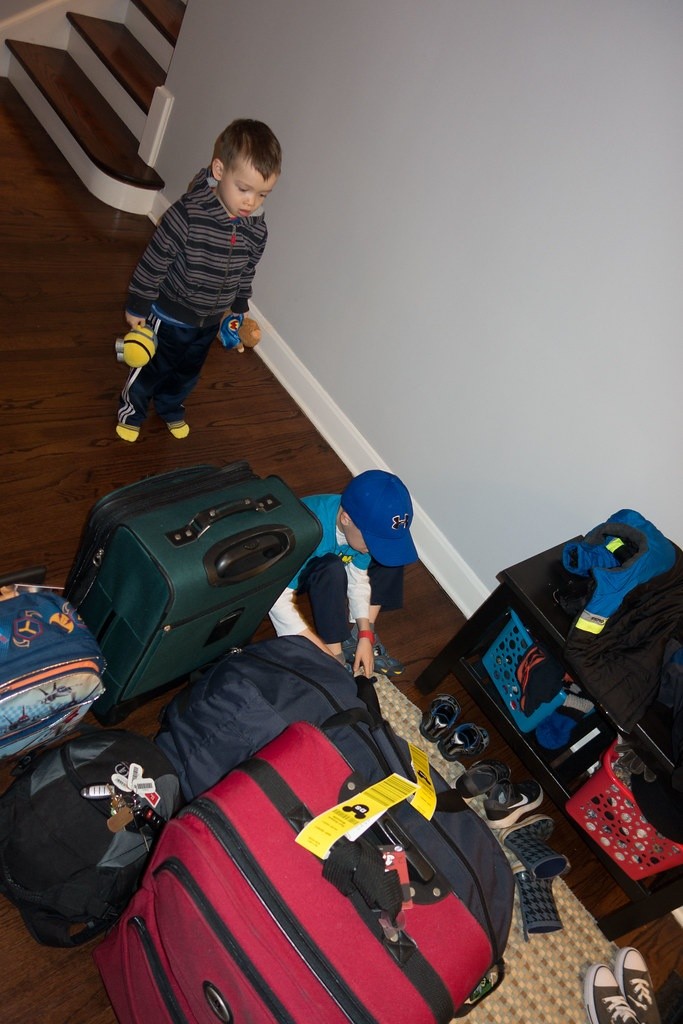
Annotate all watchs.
[357,630,376,645]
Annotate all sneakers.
[420,694,461,741]
[584,963,638,1024]
[456,761,512,797]
[345,636,405,678]
[482,778,544,829]
[437,723,488,762]
[614,946,661,1024]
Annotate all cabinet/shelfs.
[415,534,683,944]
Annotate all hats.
[341,470,418,567]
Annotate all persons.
[116,118,283,441]
[268,470,419,680]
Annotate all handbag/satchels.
[153,633,514,969]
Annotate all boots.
[500,814,567,880]
[509,855,571,941]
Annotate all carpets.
[360,665,621,1024]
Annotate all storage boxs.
[564,736,683,883]
[483,607,598,734]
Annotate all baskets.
[482,607,595,735]
[565,739,683,881]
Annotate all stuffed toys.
[116,325,158,368]
[216,310,261,353]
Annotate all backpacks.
[0,726,180,949]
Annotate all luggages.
[0,564,107,761]
[62,459,323,725]
[91,721,495,1022]
